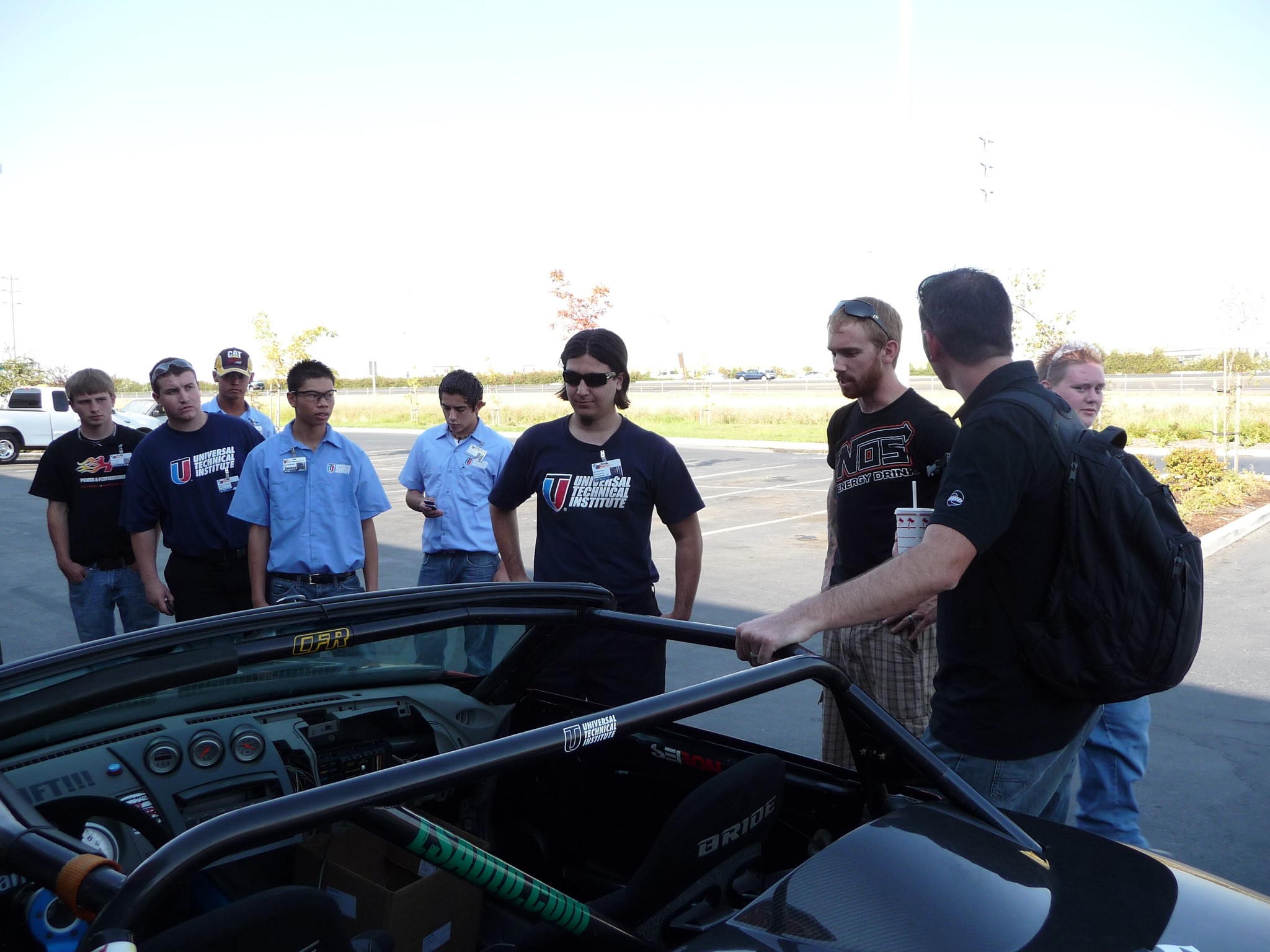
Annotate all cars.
[0,581,1270,952]
[695,372,727,379]
[799,371,831,378]
[118,397,169,426]
[247,378,265,390]
[736,369,776,382]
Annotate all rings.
[750,651,758,659]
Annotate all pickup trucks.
[0,385,161,465]
[654,371,683,379]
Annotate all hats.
[213,349,253,379]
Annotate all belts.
[200,548,249,562]
[78,555,139,573]
[439,546,469,556]
[271,569,360,586]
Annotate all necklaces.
[450,428,476,443]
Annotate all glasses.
[150,359,195,393]
[77,423,117,448]
[833,297,895,348]
[290,388,338,404]
[220,370,249,382]
[560,368,621,390]
[1044,343,1087,392]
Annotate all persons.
[817,295,961,776]
[226,358,392,661]
[118,357,266,624]
[397,369,511,675]
[488,327,708,710]
[27,368,160,645]
[733,266,1104,827]
[200,348,277,442]
[1038,342,1153,850]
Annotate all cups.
[894,507,934,555]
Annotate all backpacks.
[944,389,1207,713]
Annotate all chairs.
[455,719,787,952]
[186,885,354,950]
